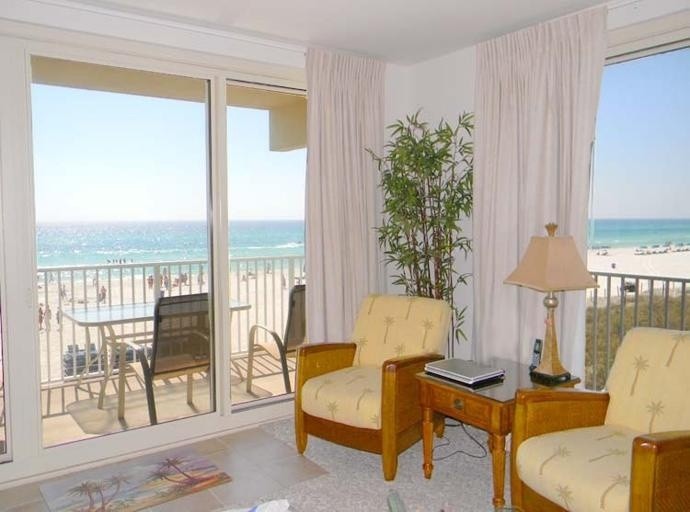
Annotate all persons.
[36,258,206,330]
[241,262,306,289]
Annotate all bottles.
[532,339,540,367]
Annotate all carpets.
[39,448,233,512]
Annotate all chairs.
[295,294,451,481]
[509,326,690,512]
[247,284,305,394]
[118,293,210,425]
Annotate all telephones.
[529,339,542,369]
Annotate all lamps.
[502,223,599,387]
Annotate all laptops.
[423,356,506,386]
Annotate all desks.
[62,298,252,408]
[414,358,580,506]
